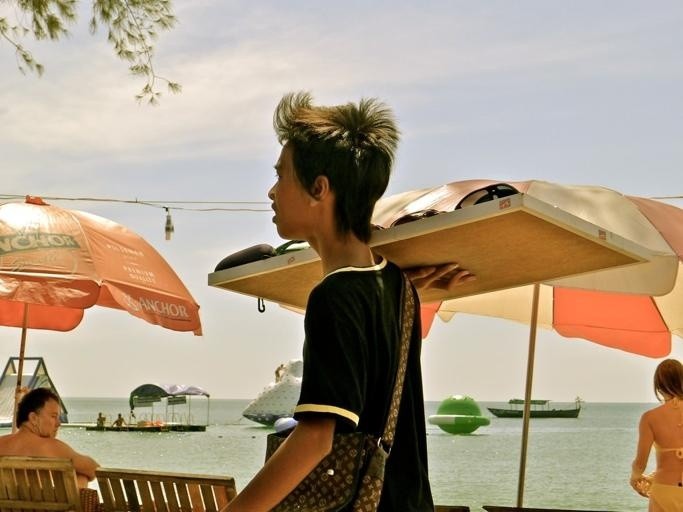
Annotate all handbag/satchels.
[214,244,279,274]
[264,424,389,511]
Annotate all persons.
[0,388,100,490]
[275,363,285,383]
[630,359,683,512]
[97,413,105,426]
[220,92,477,509]
[112,413,127,427]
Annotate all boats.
[486,396,582,419]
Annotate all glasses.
[454,184,519,212]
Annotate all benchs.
[0,456,82,512]
[95,468,237,512]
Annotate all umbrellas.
[1,195,203,435]
[279,180,682,507]
